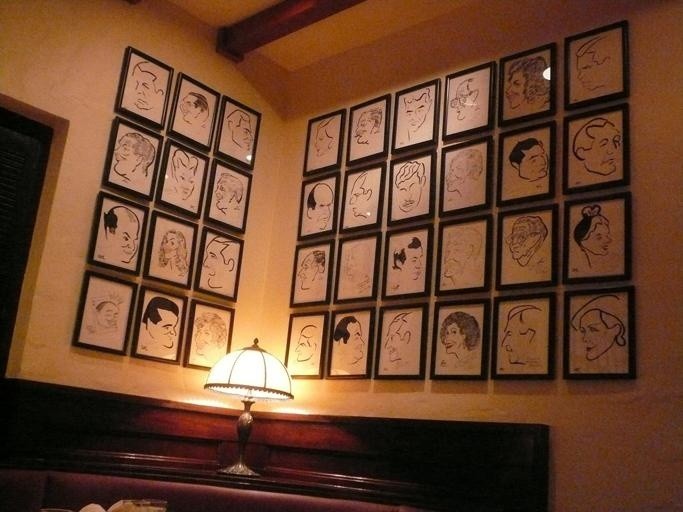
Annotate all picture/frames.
[101,46,262,236]
[71,191,245,371]
[374,60,496,383]
[490,18,639,381]
[283,94,392,380]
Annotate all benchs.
[0,467,413,511]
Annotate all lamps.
[200,337,292,480]
